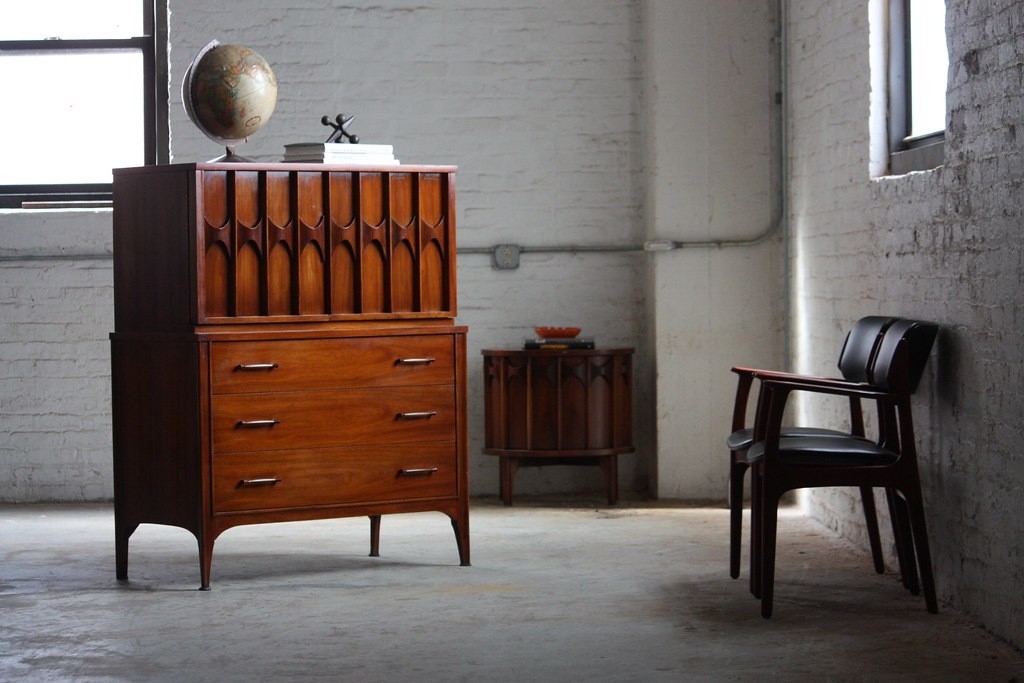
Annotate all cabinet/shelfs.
[113,161,470,591]
[482,348,636,506]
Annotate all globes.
[179,39,279,163]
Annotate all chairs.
[747,319,944,618]
[727,316,913,593]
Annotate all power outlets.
[496,244,519,270]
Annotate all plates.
[534,326,580,339]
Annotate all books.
[278,142,400,166]
